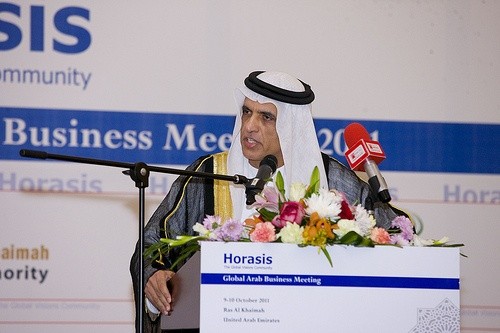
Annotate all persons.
[128,69,406,332]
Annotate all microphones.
[343,122,392,203]
[244,153,279,205]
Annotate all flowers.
[196,182,438,257]
